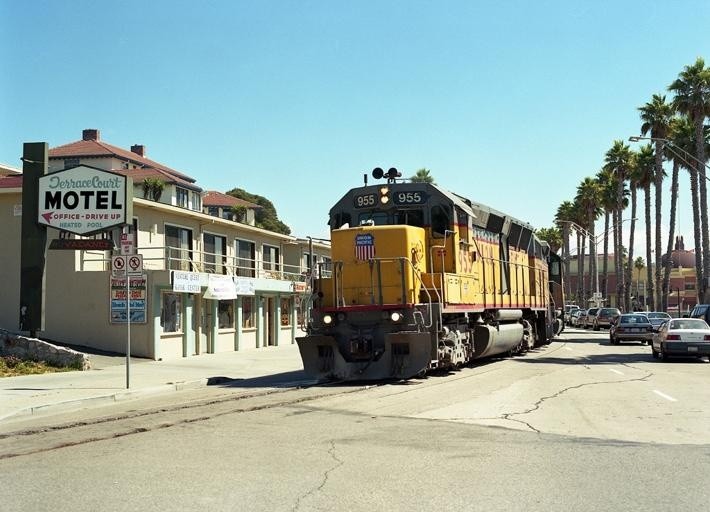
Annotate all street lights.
[628,136,710,181]
[553,217,638,307]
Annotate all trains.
[292,165,565,381]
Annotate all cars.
[563,303,710,362]
